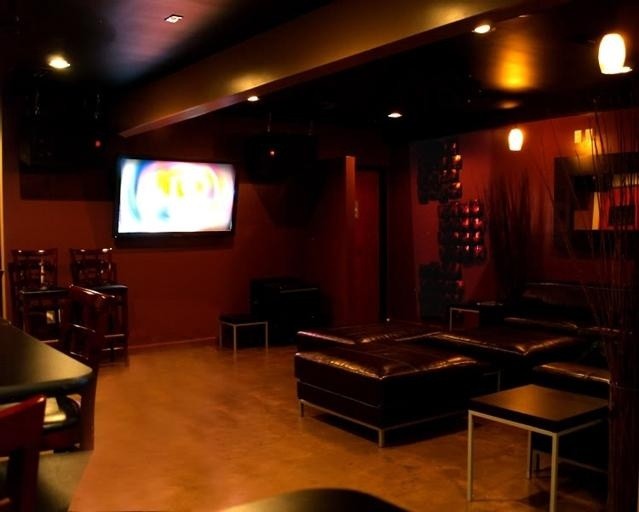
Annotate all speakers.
[15,112,109,174]
[243,131,320,183]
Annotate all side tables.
[218,315,270,350]
[468,384,609,512]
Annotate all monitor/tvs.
[113,154,240,240]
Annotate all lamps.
[508,124,524,152]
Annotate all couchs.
[478,283,638,342]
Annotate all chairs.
[0,248,129,512]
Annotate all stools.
[294,340,478,447]
[292,318,610,391]
